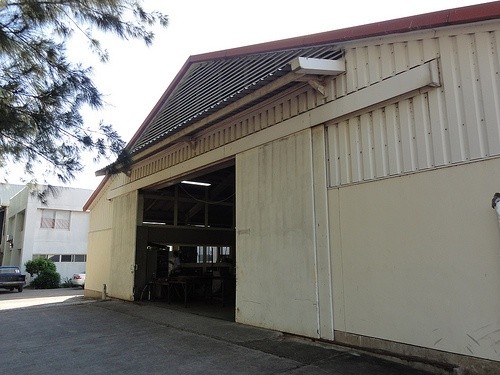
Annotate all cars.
[72,271,86,289]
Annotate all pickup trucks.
[0,266,26,292]
